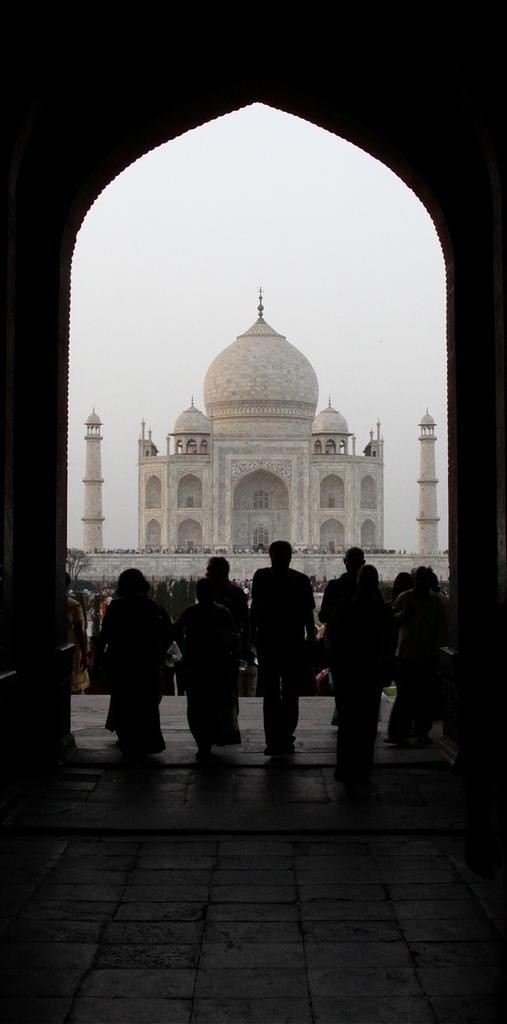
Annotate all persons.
[205,555,248,625]
[60,567,452,693]
[87,544,404,555]
[316,545,369,728]
[383,565,450,748]
[86,568,174,761]
[322,563,394,783]
[244,538,320,758]
[167,577,247,759]
